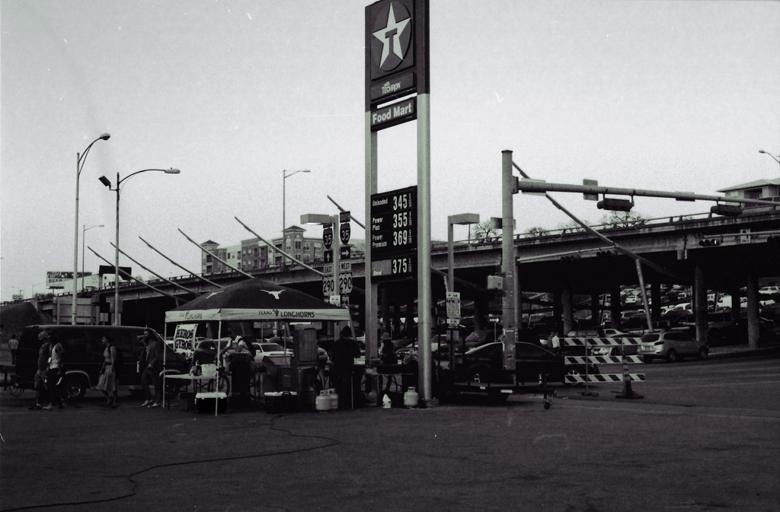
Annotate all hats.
[380,332,392,340]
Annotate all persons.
[140,328,164,409]
[379,332,398,394]
[224,327,253,376]
[46,334,65,411]
[33,329,52,411]
[96,334,120,408]
[8,334,18,365]
[331,325,361,409]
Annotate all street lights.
[757,150,780,165]
[282,168,312,264]
[70,132,180,324]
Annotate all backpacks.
[238,335,256,357]
[108,343,125,365]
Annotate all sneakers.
[29,398,160,410]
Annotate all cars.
[193,339,228,366]
[251,341,295,370]
[449,341,556,384]
[621,285,779,319]
[635,332,710,365]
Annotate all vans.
[15,324,192,401]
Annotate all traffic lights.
[698,237,721,246]
